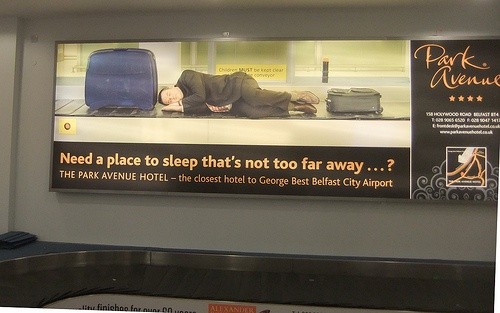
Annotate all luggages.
[325,88,383,115]
[84,47,158,110]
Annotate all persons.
[159,70,319,117]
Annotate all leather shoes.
[292,101,316,114]
[291,89,320,104]
